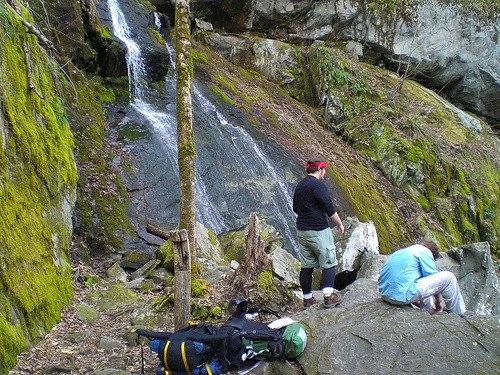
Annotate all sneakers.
[302,296,318,310]
[324,289,342,308]
[460,310,475,317]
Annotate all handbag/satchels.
[320,266,362,290]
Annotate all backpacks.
[136,308,308,374]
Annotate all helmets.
[228,297,248,317]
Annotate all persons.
[377,241,475,313]
[293,158,344,309]
